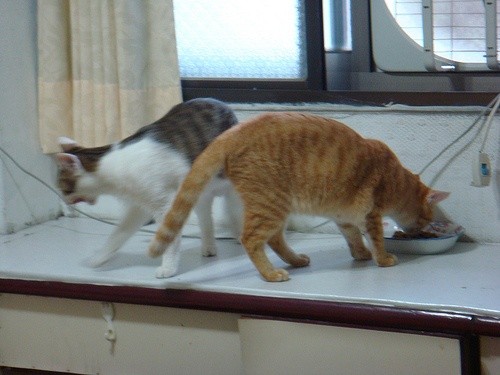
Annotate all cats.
[55,98,242,278]
[149,112,450,283]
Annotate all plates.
[362,222,465,254]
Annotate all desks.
[0,217,500,375]
